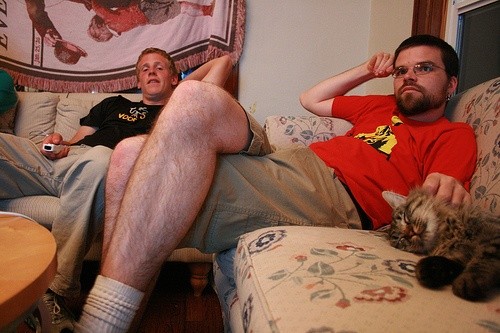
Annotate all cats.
[372,183,500,302]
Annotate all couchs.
[212,78,500,333]
[0,92,212,300]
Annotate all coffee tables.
[0,211,59,333]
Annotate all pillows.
[54,98,93,142]
[16,93,60,146]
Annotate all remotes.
[43,144,68,155]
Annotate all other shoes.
[24,291,74,333]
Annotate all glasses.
[392,61,445,78]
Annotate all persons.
[74,34,478,333]
[0,47,233,333]
[0,69,17,134]
